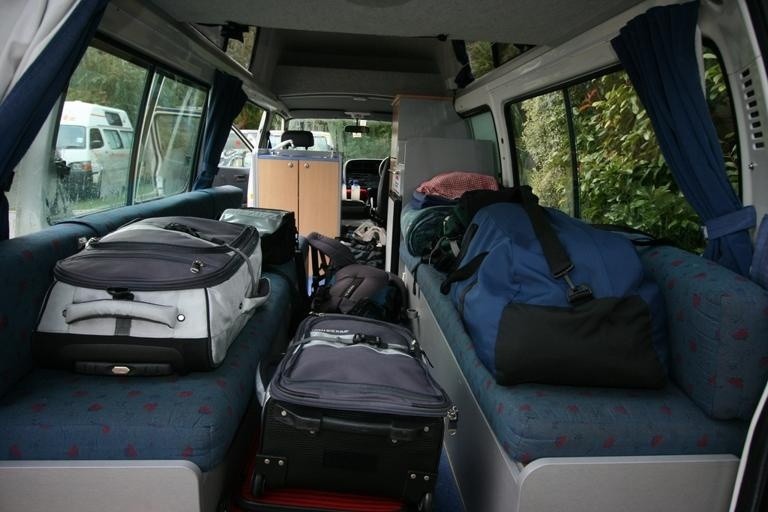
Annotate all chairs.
[369,157,390,220]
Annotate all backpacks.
[303,231,410,326]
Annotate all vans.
[55,101,134,201]
[222,129,334,158]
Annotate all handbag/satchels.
[440,201,674,392]
[216,206,301,266]
[406,185,539,295]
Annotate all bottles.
[350,180,361,200]
[342,181,347,199]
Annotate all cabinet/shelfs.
[258,149,342,277]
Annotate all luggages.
[249,311,461,512]
[35,212,271,380]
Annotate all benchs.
[0,185,309,512]
[399,231,768,512]
